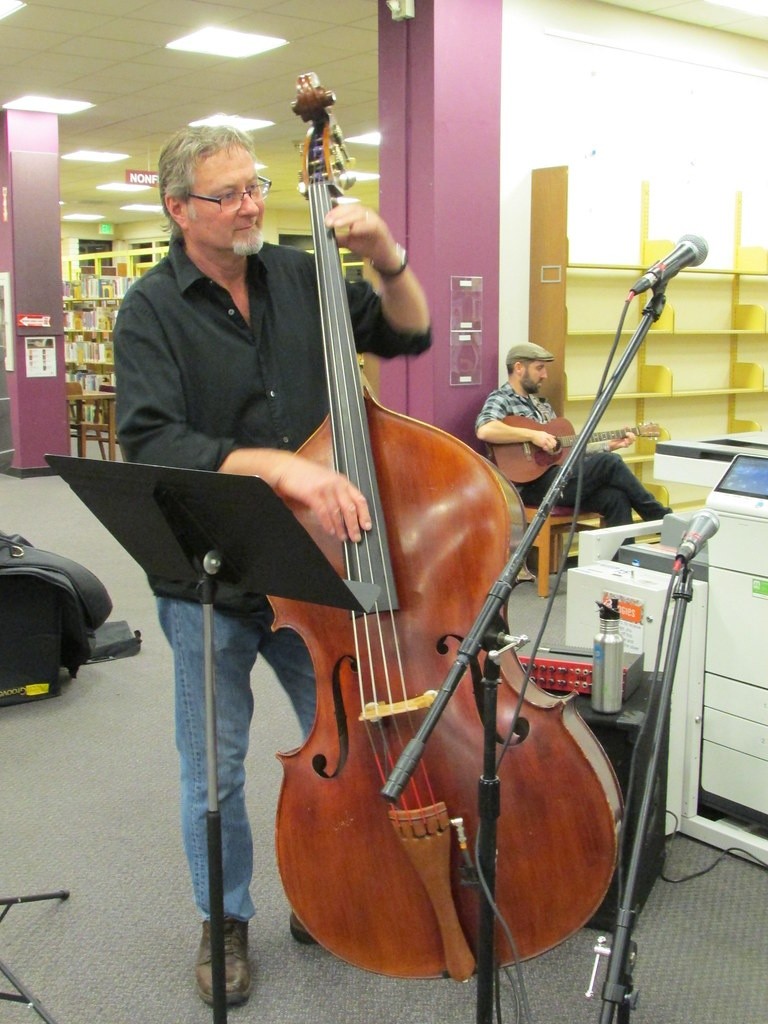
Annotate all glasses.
[184,176,273,214]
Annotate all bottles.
[590,598,624,714]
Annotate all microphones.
[672,506,721,573]
[631,234,709,295]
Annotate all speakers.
[553,668,671,932]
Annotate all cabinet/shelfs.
[60,243,170,432]
[529,165,767,556]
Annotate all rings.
[366,212,368,220]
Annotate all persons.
[113,125,433,1008]
[476,342,674,545]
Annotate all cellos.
[260,69,627,985]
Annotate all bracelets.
[369,244,408,275]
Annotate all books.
[64,341,113,362]
[66,373,116,423]
[63,274,139,298]
[63,307,118,330]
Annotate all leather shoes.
[196,915,249,1003]
[289,911,318,944]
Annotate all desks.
[65,389,116,462]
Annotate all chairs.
[486,440,607,597]
[64,382,107,460]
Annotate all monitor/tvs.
[714,455,768,500]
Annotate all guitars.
[492,415,663,484]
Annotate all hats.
[505,341,553,365]
